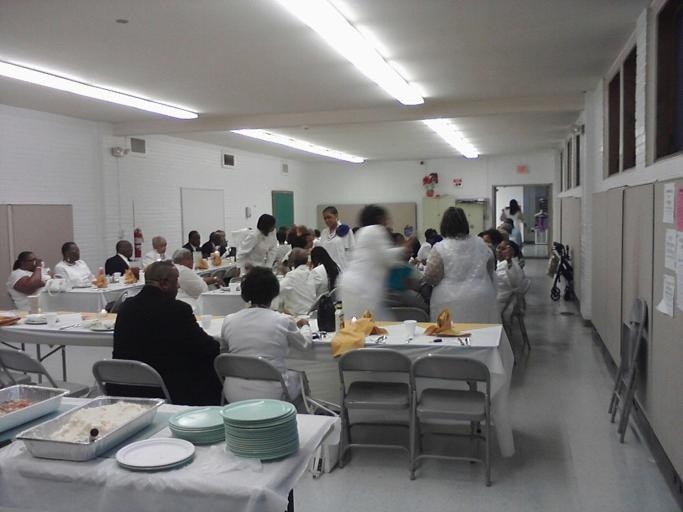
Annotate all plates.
[90,322,114,331]
[221,398,299,463]
[115,437,196,471]
[0,384,70,434]
[16,395,165,462]
[168,407,225,446]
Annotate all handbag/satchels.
[45,275,71,295]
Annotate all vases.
[427,189,434,198]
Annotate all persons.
[391,223,443,269]
[425,207,516,459]
[105,262,218,406]
[54,242,98,283]
[183,230,208,259]
[7,251,63,311]
[236,214,280,277]
[478,200,524,306]
[143,237,168,270]
[310,245,340,297]
[276,223,321,248]
[314,206,356,267]
[220,267,314,413]
[105,240,139,274]
[278,248,317,315]
[203,231,235,257]
[336,206,416,324]
[173,249,208,306]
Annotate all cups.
[402,320,417,339]
[24,318,48,325]
[200,315,212,331]
[27,295,41,315]
[229,283,239,293]
[113,272,121,284]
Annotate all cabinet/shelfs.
[453,198,487,237]
[421,193,455,245]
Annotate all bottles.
[335,300,344,331]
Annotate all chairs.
[202,270,213,281]
[503,279,534,354]
[227,265,237,281]
[213,269,226,287]
[214,353,310,416]
[337,349,415,480]
[108,289,125,314]
[411,354,492,492]
[92,361,171,405]
[608,298,647,444]
[392,306,429,323]
[0,349,89,398]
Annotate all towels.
[361,312,383,335]
[123,271,136,283]
[333,319,373,357]
[425,311,457,335]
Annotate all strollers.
[550,242,576,301]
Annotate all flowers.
[421,174,438,191]
